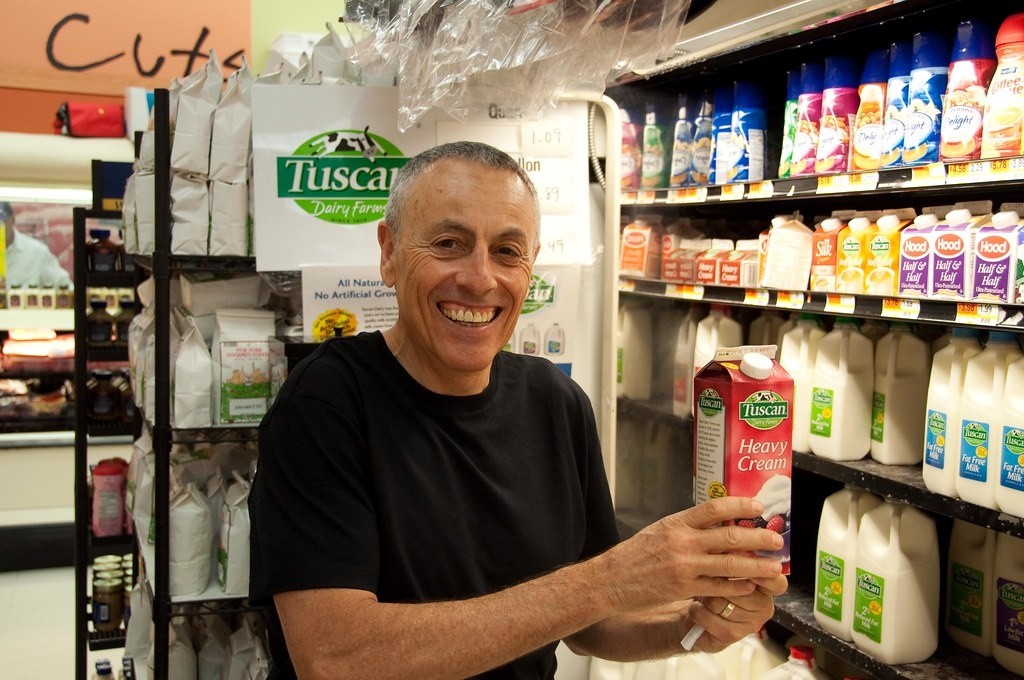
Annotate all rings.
[720,602,734,618]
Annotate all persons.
[0,201,73,292]
[252,141,787,680]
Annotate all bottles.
[93,457,132,537]
[90,656,136,680]
[85,228,141,437]
[615,295,1024,680]
